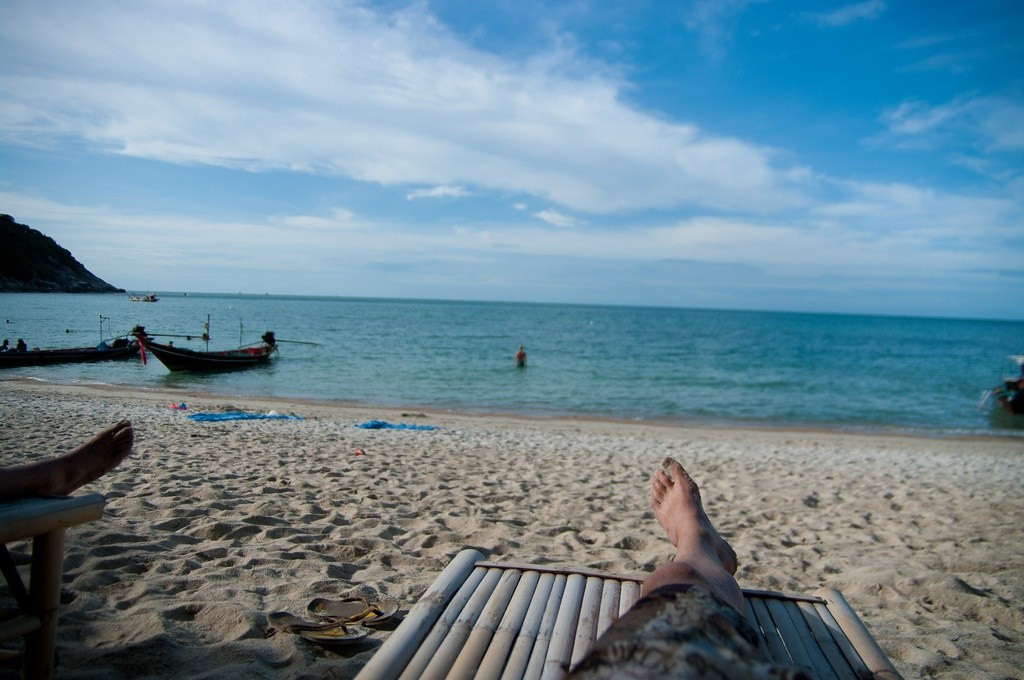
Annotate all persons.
[0,338,28,352]
[511,344,527,367]
[1,418,134,504]
[570,459,822,680]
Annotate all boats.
[1,315,145,366]
[131,293,159,302]
[131,326,277,373]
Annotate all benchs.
[0,493,106,680]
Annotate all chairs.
[352,545,903,680]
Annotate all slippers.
[307,596,401,626]
[266,611,368,642]
